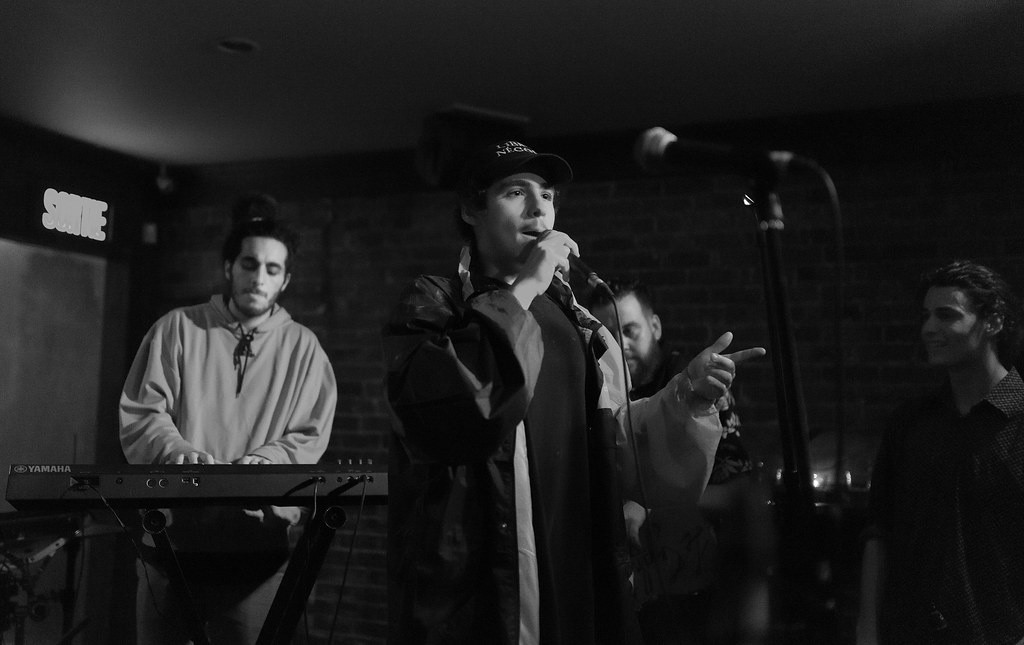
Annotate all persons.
[871,260,1024,644]
[117,217,338,645]
[582,275,755,645]
[382,135,768,645]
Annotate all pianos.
[3,454,391,507]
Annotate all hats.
[462,140,573,199]
[222,196,299,256]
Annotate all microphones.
[536,229,617,300]
[635,128,822,179]
